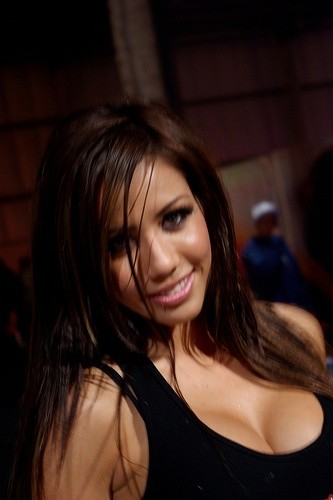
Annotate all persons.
[0,100,332,500]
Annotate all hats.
[251,201,275,219]
